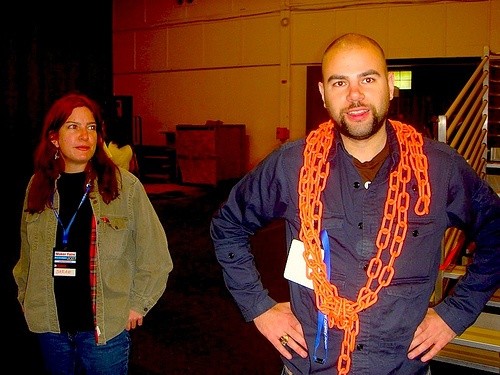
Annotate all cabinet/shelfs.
[175,124,244,187]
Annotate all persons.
[12,94,173,375]
[210,33,500,375]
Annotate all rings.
[280,335,290,346]
